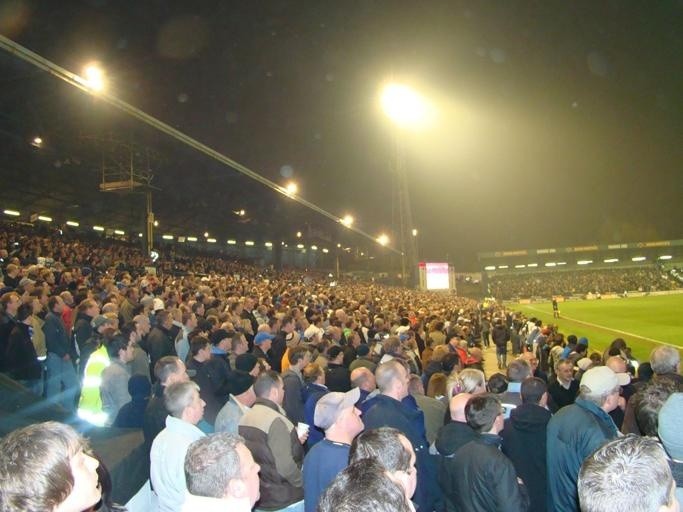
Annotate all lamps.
[527,263,538,267]
[576,259,593,265]
[557,262,567,265]
[296,231,302,238]
[153,219,160,228]
[376,234,389,246]
[5,209,20,217]
[38,216,53,222]
[515,264,525,268]
[544,262,556,267]
[285,180,299,196]
[162,234,174,239]
[322,248,329,253]
[412,228,417,237]
[338,215,354,228]
[34,137,41,144]
[632,256,646,261]
[94,225,104,231]
[226,239,236,244]
[187,237,198,241]
[265,242,273,246]
[484,265,495,270]
[281,241,288,248]
[498,265,508,268]
[337,243,341,247]
[604,258,619,263]
[297,244,303,248]
[80,61,108,93]
[67,221,79,227]
[207,238,216,242]
[114,230,125,235]
[139,232,142,236]
[233,208,246,217]
[659,255,672,260]
[245,240,254,245]
[311,245,318,250]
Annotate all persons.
[282,346,309,423]
[214,371,257,433]
[435,394,476,458]
[487,262,683,304]
[318,459,416,512]
[183,432,261,512]
[43,294,79,413]
[453,368,484,397]
[545,359,579,415]
[553,297,560,318]
[363,361,430,455]
[576,432,679,511]
[548,333,565,377]
[441,395,530,511]
[0,292,21,373]
[491,319,509,369]
[568,344,588,365]
[658,390,683,511]
[120,289,140,327]
[143,355,191,494]
[560,335,588,360]
[349,427,419,501]
[0,422,105,512]
[620,362,654,414]
[113,374,151,428]
[574,352,601,382]
[60,290,75,339]
[120,320,150,378]
[489,302,510,326]
[350,366,377,405]
[0,224,179,314]
[7,303,42,379]
[605,357,635,428]
[519,351,547,380]
[609,348,620,357]
[75,300,101,348]
[406,374,446,447]
[149,380,207,512]
[499,376,553,511]
[323,345,351,392]
[186,336,219,424]
[545,366,623,511]
[100,336,135,428]
[506,359,535,392]
[481,315,490,349]
[603,339,627,366]
[486,372,508,394]
[427,373,448,400]
[79,315,112,392]
[300,387,366,512]
[302,363,330,454]
[620,347,639,370]
[509,311,558,355]
[145,311,177,362]
[23,296,47,371]
[620,344,680,438]
[100,327,116,347]
[237,370,304,511]
[174,253,485,374]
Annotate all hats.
[89,312,118,329]
[657,392,683,461]
[355,344,369,356]
[579,365,631,396]
[16,303,33,320]
[313,386,361,430]
[140,280,151,288]
[116,282,127,289]
[210,329,234,344]
[326,345,345,361]
[396,326,410,336]
[19,278,36,286]
[577,358,593,371]
[304,326,319,341]
[221,369,256,395]
[81,267,92,275]
[285,330,302,348]
[399,335,410,340]
[579,337,587,346]
[254,332,277,344]
[235,354,258,372]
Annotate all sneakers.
[497,360,502,369]
[502,363,508,368]
[485,346,487,350]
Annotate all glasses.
[556,367,574,374]
[497,407,506,416]
